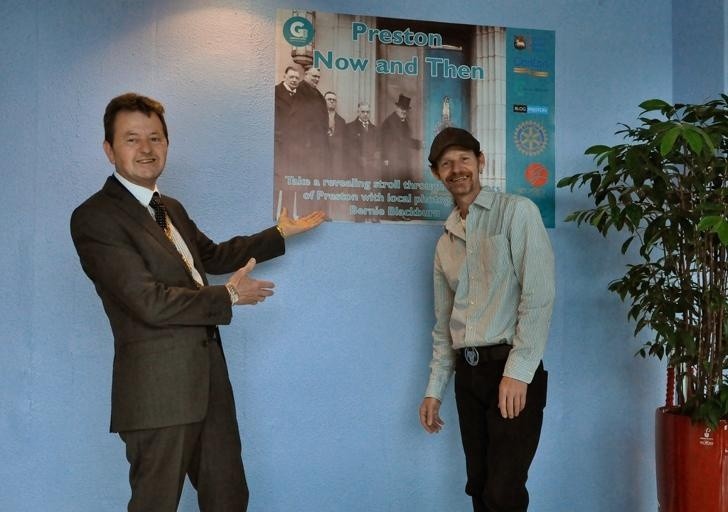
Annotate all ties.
[149,193,166,228]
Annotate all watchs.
[225,283,239,307]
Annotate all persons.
[418,126,556,512]
[276,64,424,223]
[69,92,326,512]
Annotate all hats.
[394,94,412,111]
[428,127,480,164]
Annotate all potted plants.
[555,92,728,512]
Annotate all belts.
[454,344,510,367]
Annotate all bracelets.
[276,226,288,239]
